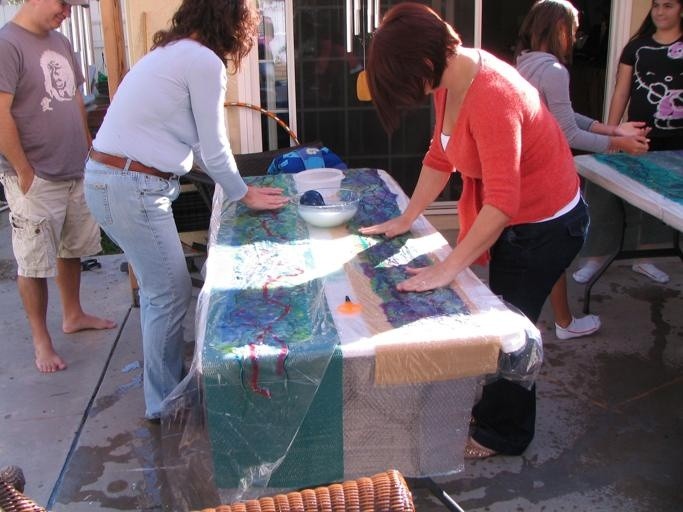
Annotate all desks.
[196,167,539,510]
[573,152,683,313]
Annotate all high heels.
[462,436,499,459]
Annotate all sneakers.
[555,313,601,340]
[572,258,610,283]
[631,263,669,283]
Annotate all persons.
[572,0,683,284]
[357,2,591,459]
[83,0,290,426]
[514,0,652,340]
[0,0,117,372]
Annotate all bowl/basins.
[288,187,363,228]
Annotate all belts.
[87,147,177,181]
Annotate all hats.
[60,1,92,8]
